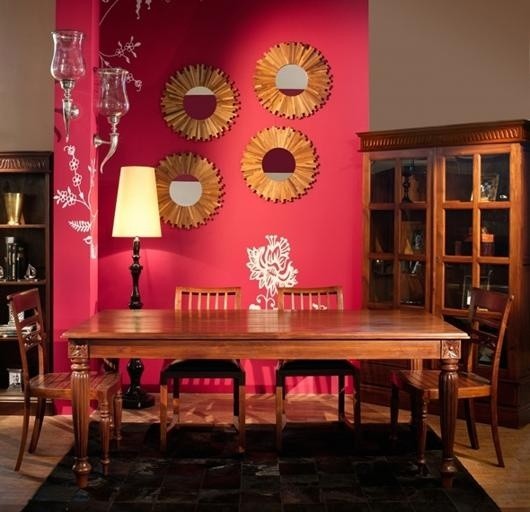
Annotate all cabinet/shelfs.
[355,118,530,430]
[1,149,56,418]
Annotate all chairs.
[9,287,126,489]
[158,286,246,446]
[273,285,360,439]
[388,285,516,480]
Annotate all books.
[0,232,36,340]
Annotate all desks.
[58,308,472,488]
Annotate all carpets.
[19,418,502,512]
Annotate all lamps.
[110,164,166,410]
[48,27,87,146]
[93,65,131,175]
